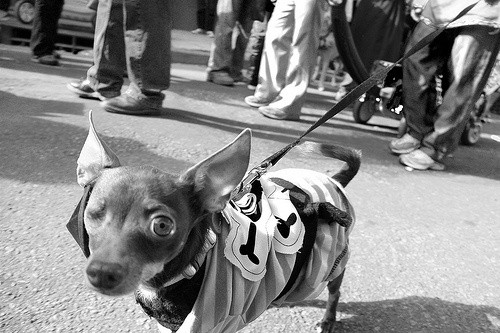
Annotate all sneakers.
[100,93,161,115]
[227,70,247,86]
[399,149,445,171]
[258,106,299,121]
[67,80,122,100]
[206,72,234,85]
[388,133,422,154]
[244,95,271,107]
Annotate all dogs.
[76,109,362,333]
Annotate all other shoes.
[29,54,59,66]
[49,51,62,59]
[207,30,214,37]
[192,28,204,34]
[247,80,258,90]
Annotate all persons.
[244,0,343,120]
[30,0,64,65]
[67,0,172,116]
[389,0,500,170]
[190,0,217,37]
[206,0,276,90]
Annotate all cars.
[0,0,100,26]
